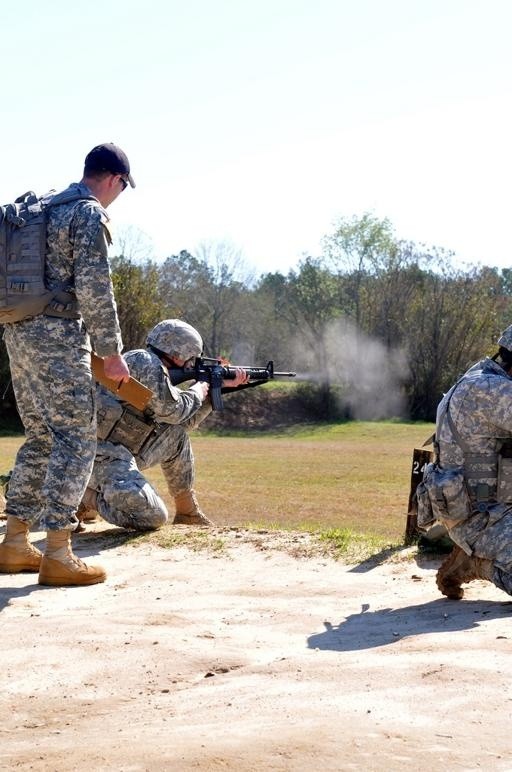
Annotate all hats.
[85,142,136,188]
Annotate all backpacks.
[0,187,100,325]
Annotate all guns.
[166,358,297,412]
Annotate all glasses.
[110,171,128,191]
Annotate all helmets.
[498,324,512,352]
[144,318,203,362]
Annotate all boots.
[0,514,43,573]
[71,488,98,533]
[174,490,216,528]
[38,530,106,586]
[436,545,494,600]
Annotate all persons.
[0,141,138,583]
[416,324,512,600]
[74,320,251,533]
